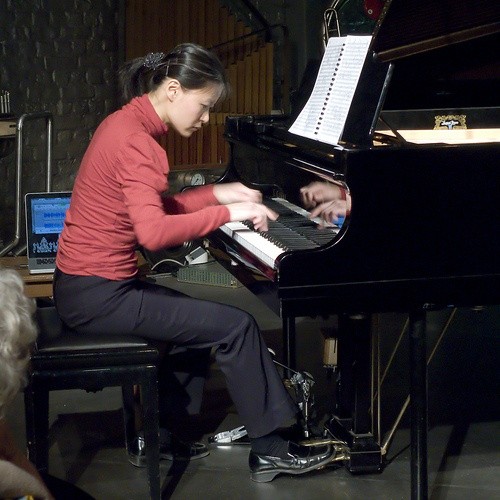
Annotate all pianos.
[181,114,500,476]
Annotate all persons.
[50,41,335,484]
[0,269,58,500]
[299,180,352,230]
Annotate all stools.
[24,305,160,500]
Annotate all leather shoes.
[125,428,211,468]
[248,441,336,482]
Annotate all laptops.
[24,191,73,275]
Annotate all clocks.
[190,173,205,186]
